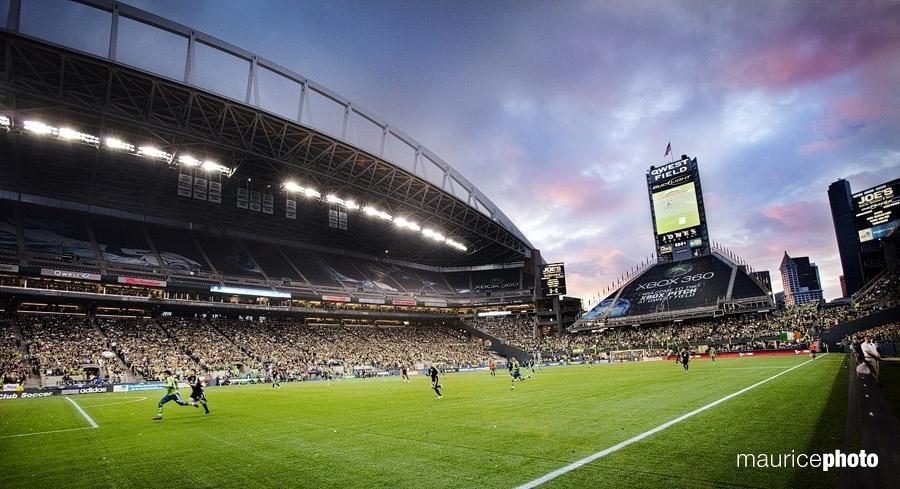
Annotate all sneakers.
[439,383,443,389]
[193,402,199,408]
[150,414,163,420]
[437,395,443,399]
[204,410,210,415]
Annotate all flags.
[664,143,672,157]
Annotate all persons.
[0,277,900,420]
